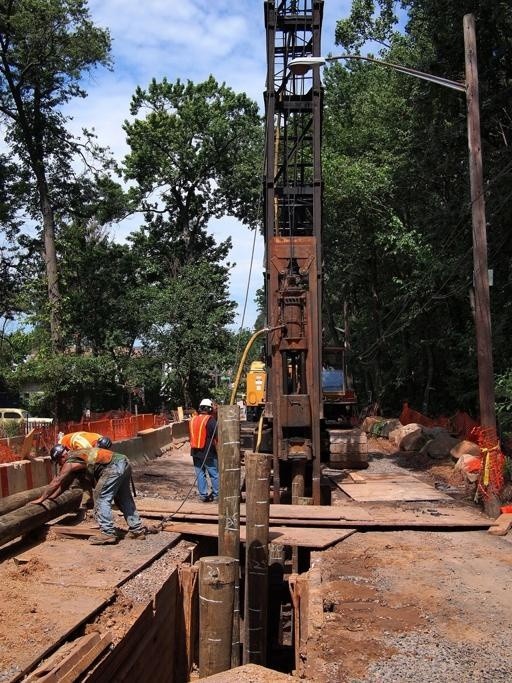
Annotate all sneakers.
[89,531,116,543]
[199,495,220,504]
[120,530,148,540]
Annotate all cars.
[0,407,53,427]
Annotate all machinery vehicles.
[238,0,367,468]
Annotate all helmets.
[199,398,213,406]
[51,443,66,460]
[98,436,112,448]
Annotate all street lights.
[288,14,502,518]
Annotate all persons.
[187,398,221,502]
[25,443,147,546]
[56,430,113,489]
